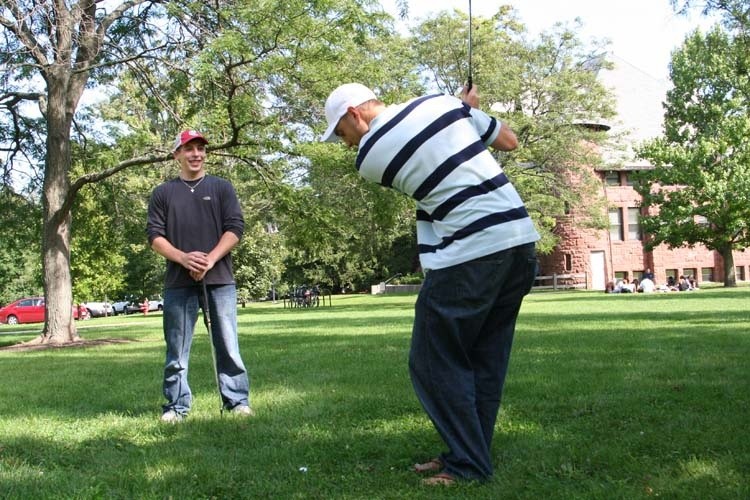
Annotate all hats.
[174,130,209,150]
[320,83,378,141]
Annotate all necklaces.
[181,176,205,192]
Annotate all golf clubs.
[466,0,473,94]
[202,274,224,420]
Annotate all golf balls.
[300,467,307,473]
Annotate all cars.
[0,296,166,325]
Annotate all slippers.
[424,473,461,487]
[414,461,450,472]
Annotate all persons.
[145,128,255,422]
[605,268,692,293]
[320,80,542,486]
[302,287,311,307]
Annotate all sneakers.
[230,404,251,415]
[163,410,183,422]
